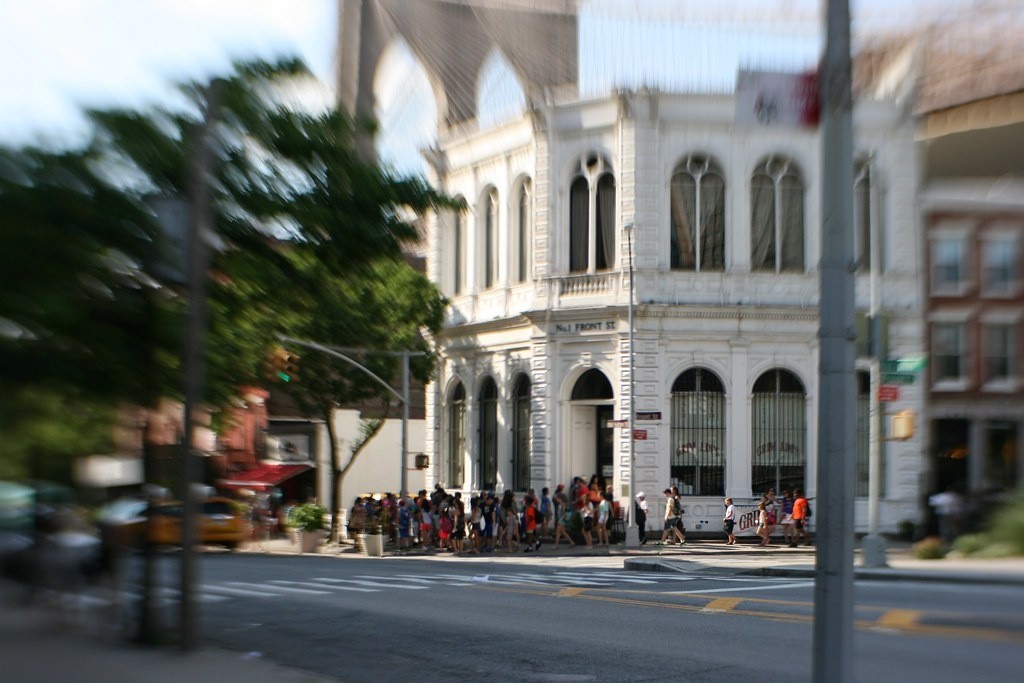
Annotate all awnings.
[215,464,309,491]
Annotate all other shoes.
[486,548,493,552]
[789,542,797,548]
[679,538,687,546]
[804,541,811,545]
[760,538,769,546]
[535,542,541,551]
[523,548,533,552]
[453,550,463,555]
[653,541,663,545]
[479,547,483,553]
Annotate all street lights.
[623,221,641,550]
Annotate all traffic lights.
[271,348,294,386]
[415,455,429,471]
[285,350,302,383]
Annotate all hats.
[557,484,564,490]
[636,491,645,498]
[663,489,671,493]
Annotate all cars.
[92,486,254,554]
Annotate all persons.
[654,486,688,546]
[722,498,737,545]
[626,491,649,545]
[286,501,299,526]
[756,487,782,547]
[787,488,812,548]
[777,489,796,544]
[347,475,616,555]
[94,486,128,625]
[247,499,262,552]
